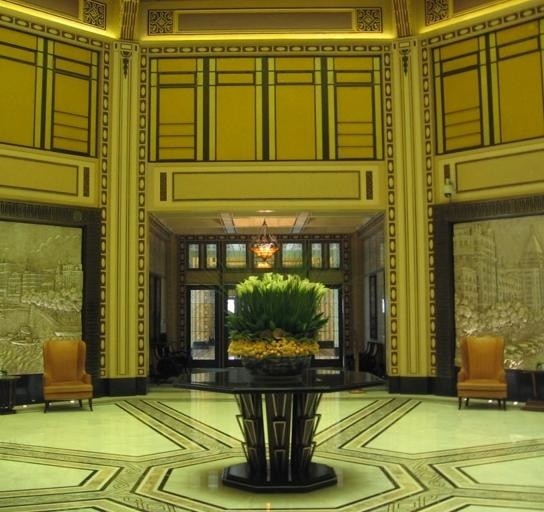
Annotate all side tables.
[0,375,22,413]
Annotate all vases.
[241,354,310,376]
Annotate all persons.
[154,332,169,372]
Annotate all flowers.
[221,272,332,362]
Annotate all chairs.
[456,336,509,410]
[42,339,94,413]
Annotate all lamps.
[249,219,280,261]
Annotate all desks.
[172,366,384,494]
